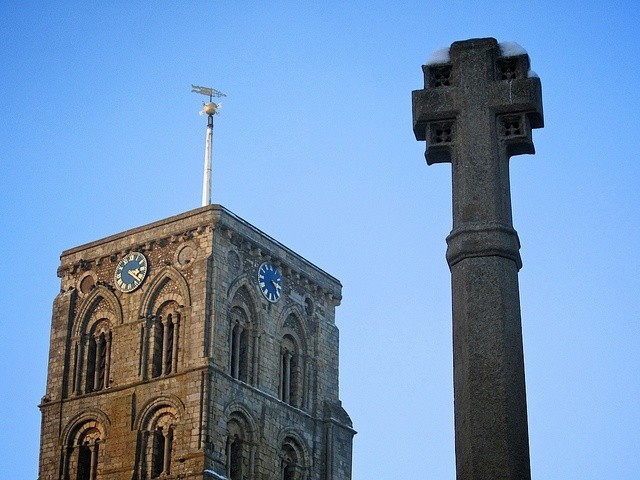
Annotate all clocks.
[257,261,283,303]
[113,251,148,293]
